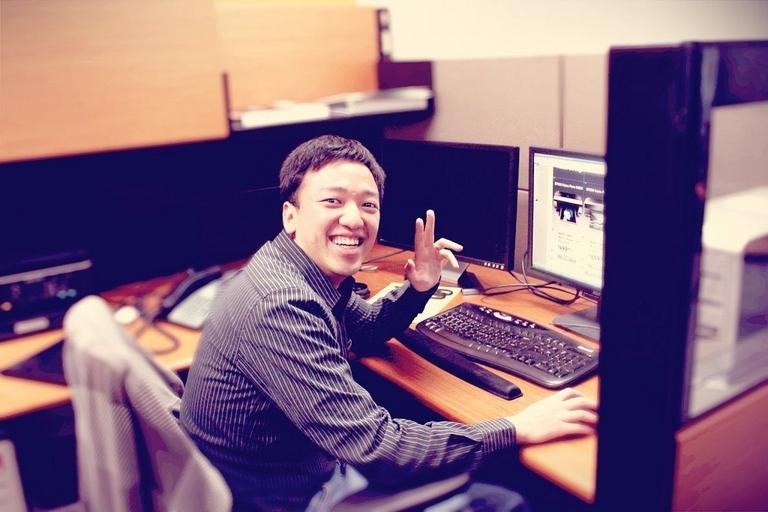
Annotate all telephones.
[161,266,226,329]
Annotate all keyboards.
[415,301,599,389]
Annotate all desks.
[0,231,768,512]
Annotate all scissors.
[430,287,454,303]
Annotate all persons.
[180,136,599,512]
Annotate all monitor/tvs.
[364,137,520,284]
[526,145,606,341]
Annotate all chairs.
[57,293,525,512]
